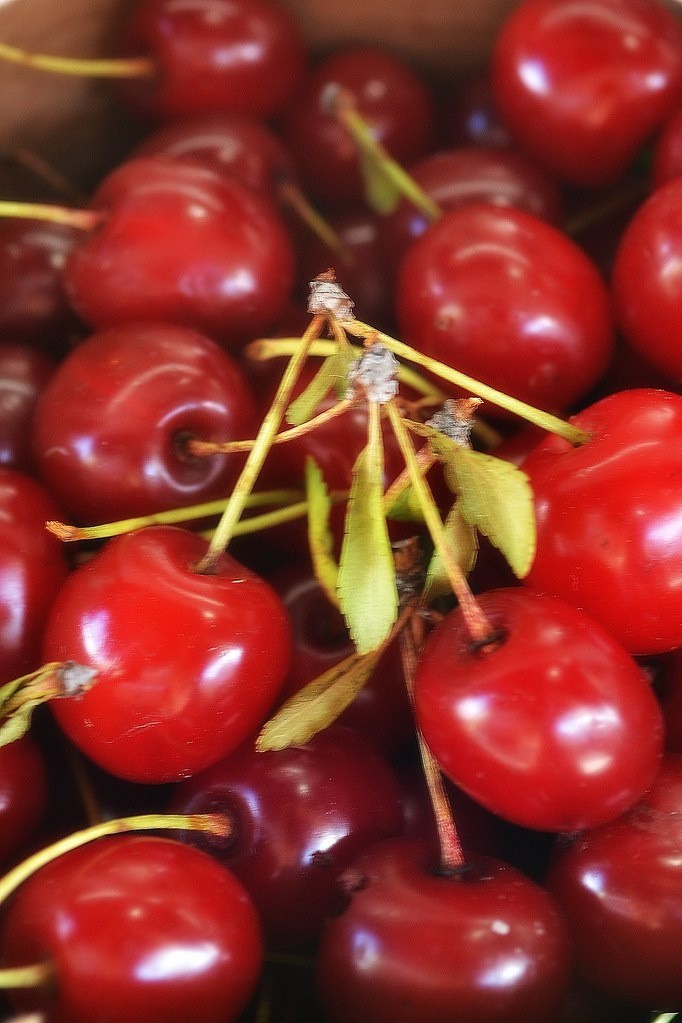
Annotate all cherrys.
[0,0,679,1021]
